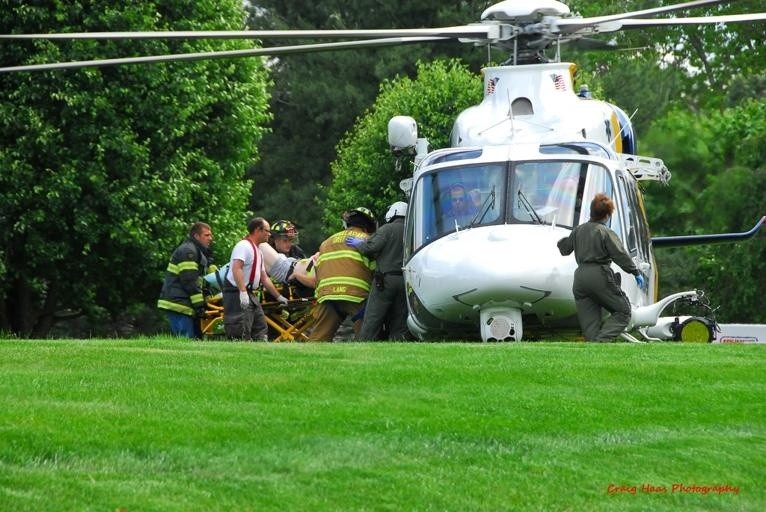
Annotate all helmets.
[270,219,298,241]
[346,206,378,235]
[385,200,409,223]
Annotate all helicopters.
[0,0,765,345]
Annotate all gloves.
[197,308,206,319]
[276,294,288,308]
[635,274,646,286]
[239,289,250,310]
[345,236,367,249]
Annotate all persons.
[439,179,484,221]
[343,200,409,342]
[267,219,311,260]
[555,192,644,342]
[308,205,378,342]
[155,220,213,341]
[221,215,288,343]
[200,242,317,291]
